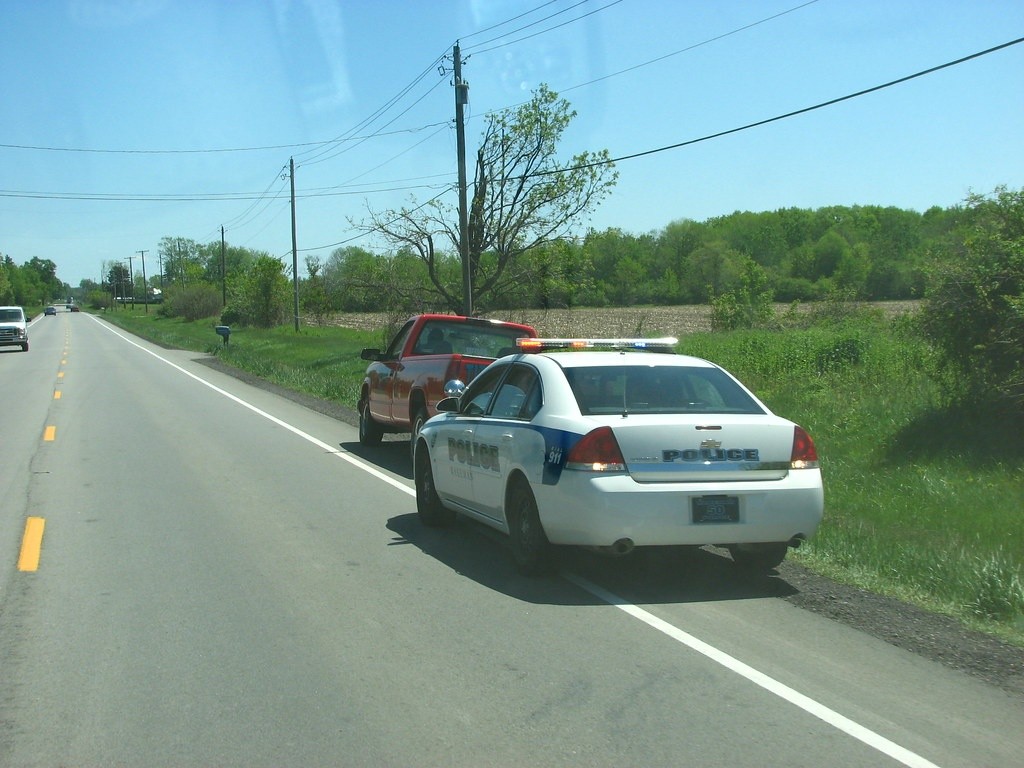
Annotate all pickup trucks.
[357,314,537,446]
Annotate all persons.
[418,328,444,350]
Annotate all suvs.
[0,306,31,351]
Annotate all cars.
[44,307,56,316]
[70,305,79,312]
[414,336,825,575]
